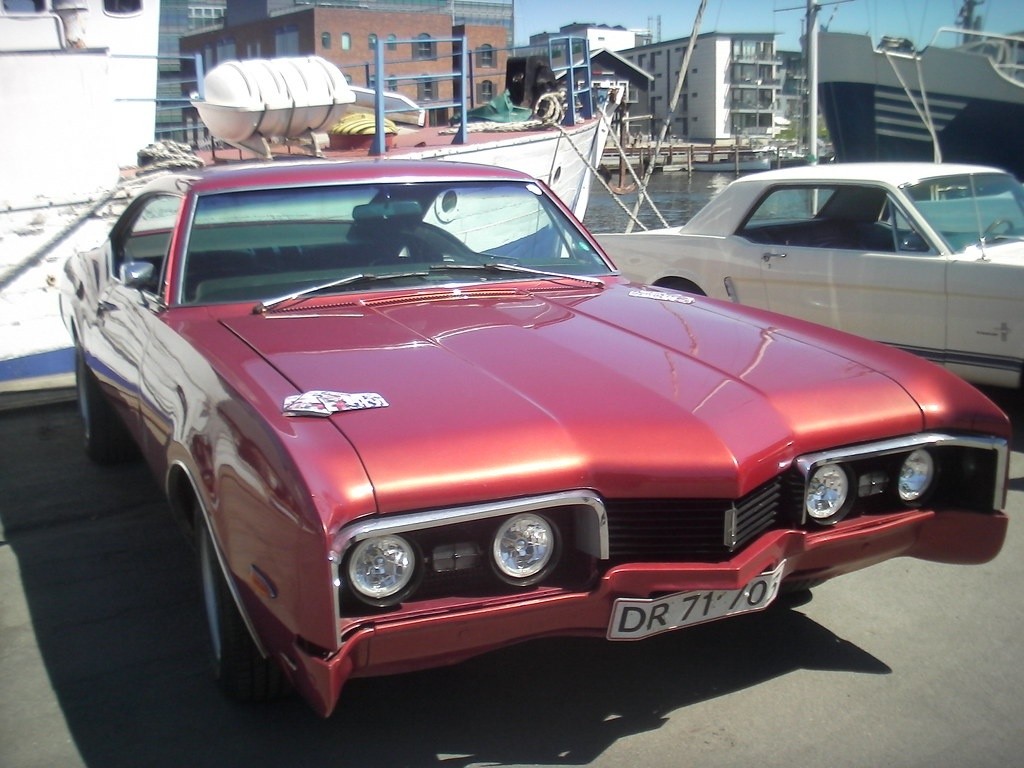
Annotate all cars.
[587,160,1022,386]
[68,158,1018,721]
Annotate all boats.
[690,150,774,172]
[803,0,1023,201]
[1,1,627,400]
